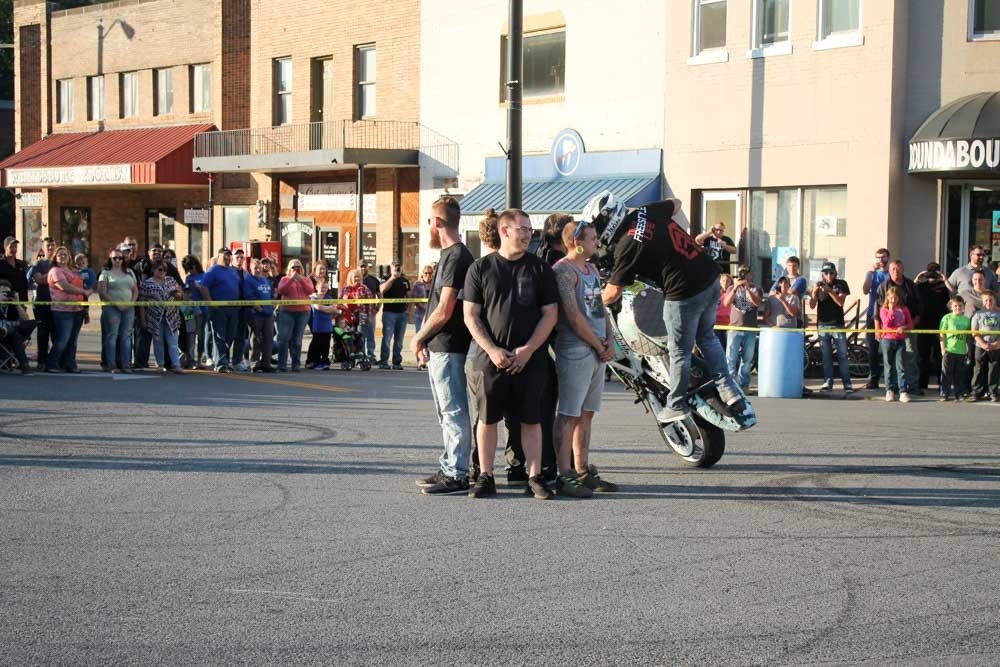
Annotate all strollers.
[323,304,371,371]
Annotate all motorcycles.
[600,268,757,468]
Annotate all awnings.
[458,172,661,212]
[908,91,1000,179]
[0,121,215,189]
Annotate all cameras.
[817,280,825,288]
[923,271,939,279]
[737,267,749,281]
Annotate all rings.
[517,365,521,368]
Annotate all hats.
[820,262,835,273]
[151,243,162,248]
[233,248,245,255]
[3,236,19,248]
[358,259,368,267]
[392,259,401,266]
[218,247,232,255]
[115,243,131,252]
[778,275,790,285]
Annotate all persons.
[0,222,999,404]
[412,194,747,495]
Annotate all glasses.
[427,216,436,225]
[973,253,985,256]
[127,243,136,246]
[157,268,167,272]
[512,226,534,234]
[823,270,834,275]
[575,220,588,237]
[38,255,44,257]
[112,256,124,261]
[291,266,301,269]
[424,272,432,275]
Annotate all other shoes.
[802,378,1000,405]
[22,356,427,377]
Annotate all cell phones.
[776,286,781,294]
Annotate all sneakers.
[716,383,741,407]
[656,406,692,425]
[414,462,620,500]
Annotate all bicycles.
[804,332,871,378]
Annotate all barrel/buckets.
[758,328,805,398]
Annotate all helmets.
[573,190,629,246]
